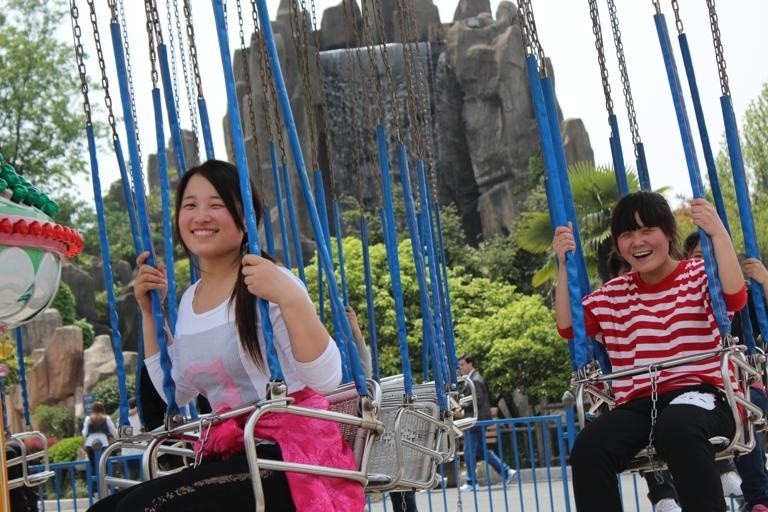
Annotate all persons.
[81,400,121,500]
[605,249,745,511]
[683,229,768,512]
[345,304,373,378]
[116,396,148,456]
[550,190,749,512]
[454,353,517,492]
[85,157,345,512]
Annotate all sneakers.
[459,483,478,492]
[505,469,516,485]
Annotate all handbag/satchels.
[75,449,88,471]
